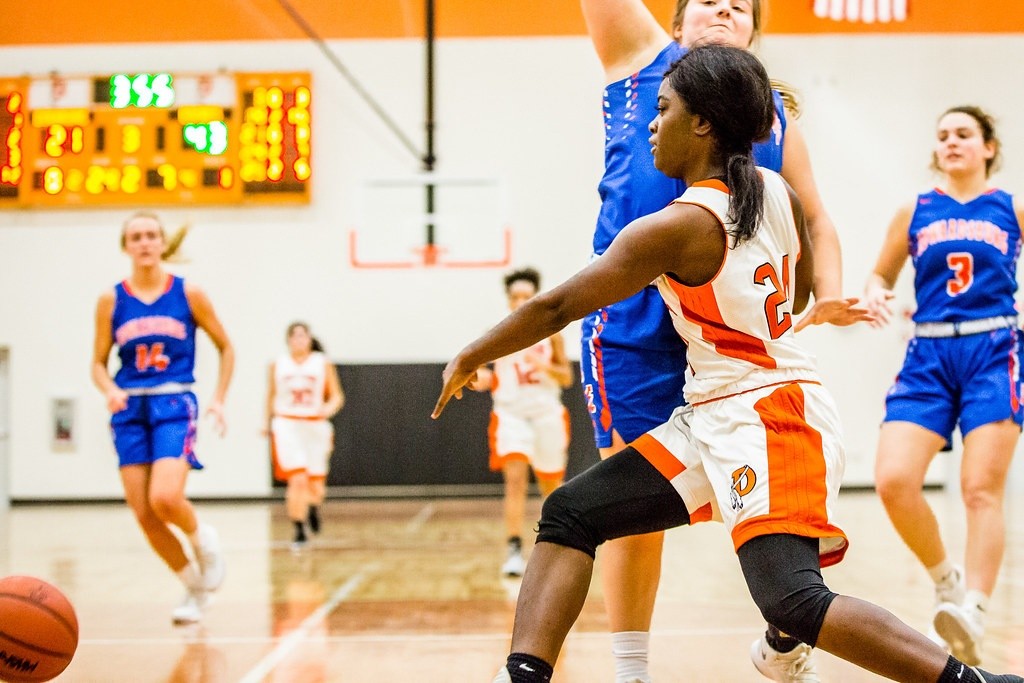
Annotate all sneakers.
[175,591,203,624]
[936,602,983,665]
[292,520,308,545]
[502,552,527,577]
[935,566,964,608]
[192,531,223,585]
[309,507,321,532]
[750,633,819,683]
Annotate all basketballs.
[0,576,80,683]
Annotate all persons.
[869,106,1021,668]
[471,269,575,580]
[581,1,870,679]
[92,208,232,624]
[267,323,344,553]
[427,38,1021,683]
